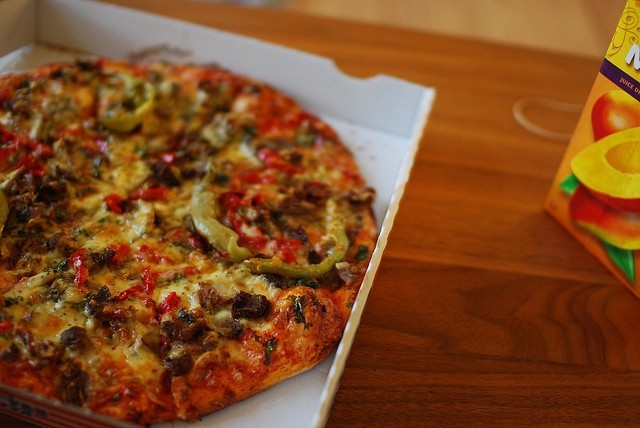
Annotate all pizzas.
[0,54,380,426]
